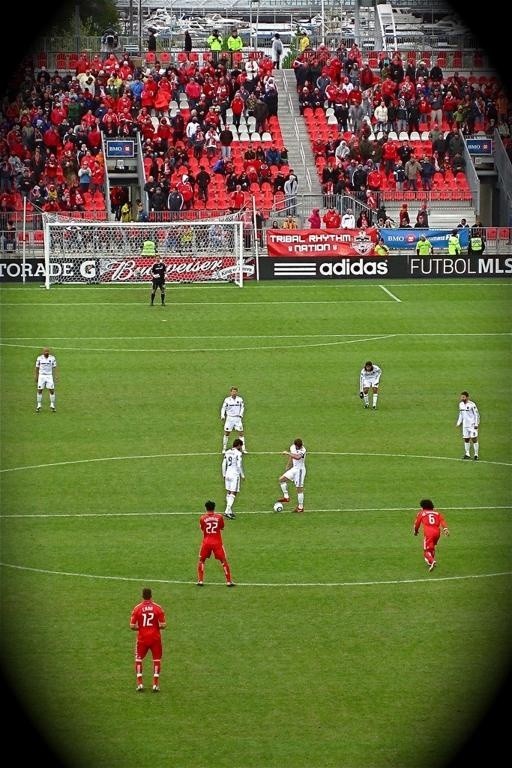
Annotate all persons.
[220,438,246,521]
[275,437,307,514]
[33,347,59,415]
[273,34,283,69]
[148,255,167,307]
[1,53,298,253]
[294,43,510,210]
[207,29,243,59]
[184,30,192,52]
[196,499,237,587]
[301,32,310,51]
[359,361,382,409]
[412,498,450,573]
[219,387,250,455]
[148,30,156,51]
[127,587,168,693]
[308,202,487,255]
[455,389,482,461]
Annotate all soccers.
[274,503,284,513]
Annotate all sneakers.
[372,406,376,409]
[136,684,143,691]
[429,561,436,571]
[197,448,304,587]
[472,456,479,461]
[152,685,160,692]
[363,403,370,408]
[50,405,56,412]
[464,455,471,459]
[36,406,41,411]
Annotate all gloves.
[359,392,364,399]
